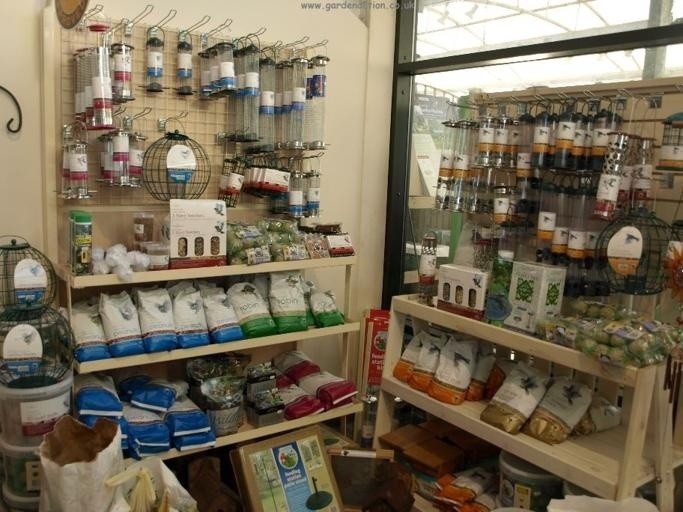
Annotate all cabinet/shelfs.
[49,255,364,491]
[372,292,683,512]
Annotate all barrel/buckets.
[1,362,72,445]
[0,441,70,496]
[2,484,42,511]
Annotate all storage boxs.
[229,424,344,512]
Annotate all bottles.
[288,169,305,218]
[69,210,87,263]
[140,241,163,255]
[73,214,93,276]
[433,111,655,298]
[132,213,154,251]
[68,137,94,200]
[419,231,438,305]
[147,244,169,270]
[97,129,148,191]
[198,42,330,151]
[271,192,288,213]
[144,36,164,93]
[306,169,321,218]
[175,41,194,96]
[73,18,136,131]
[58,144,77,200]
